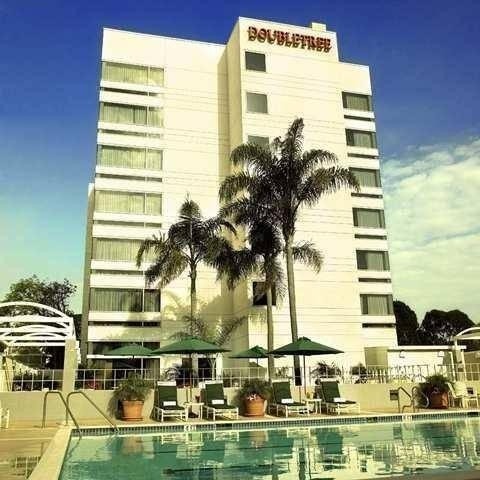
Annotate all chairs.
[151,379,186,422]
[199,379,240,420]
[452,380,479,409]
[267,382,311,417]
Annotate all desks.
[181,401,204,421]
[302,397,323,415]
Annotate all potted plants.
[237,377,273,416]
[420,374,452,409]
[114,370,150,422]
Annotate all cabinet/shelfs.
[314,377,361,415]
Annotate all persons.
[229,457,290,476]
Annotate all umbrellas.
[268,336,344,404]
[104,342,164,369]
[228,346,284,382]
[150,337,231,412]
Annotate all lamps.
[437,350,448,356]
[399,350,406,359]
